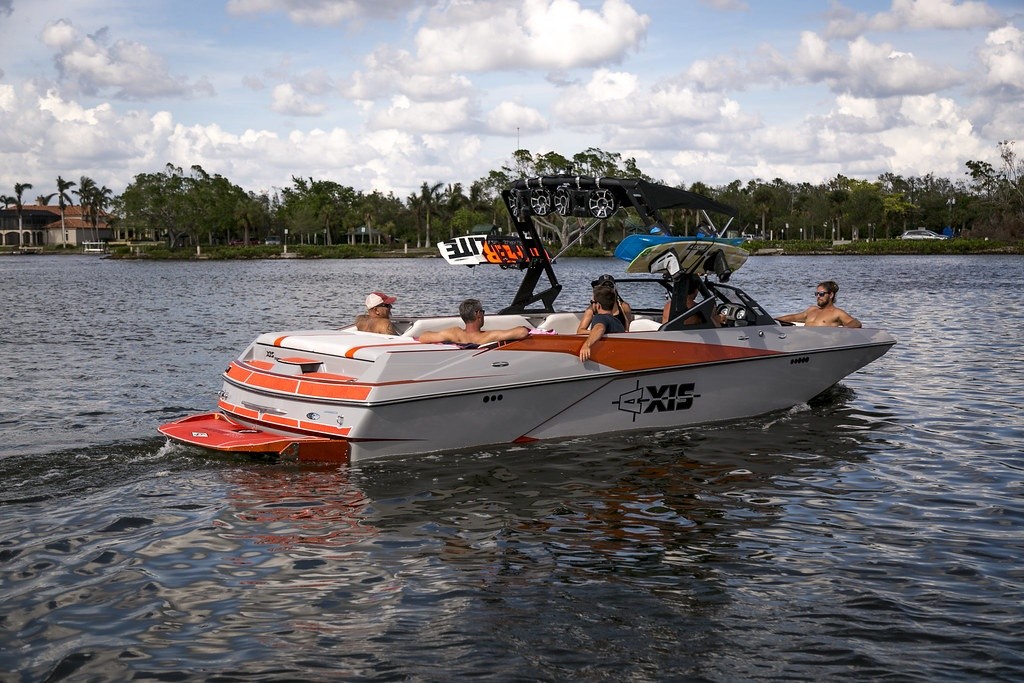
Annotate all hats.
[366,292,397,310]
[599,274,615,284]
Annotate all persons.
[577,273,632,362]
[774,281,862,328]
[356,292,400,334]
[418,299,528,344]
[662,275,726,327]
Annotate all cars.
[900,229,948,240]
[264,235,282,246]
[229,235,262,246]
[743,234,757,241]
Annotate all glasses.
[475,310,485,315]
[378,303,392,308]
[590,299,597,305]
[815,291,831,296]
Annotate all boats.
[158,174,898,463]
